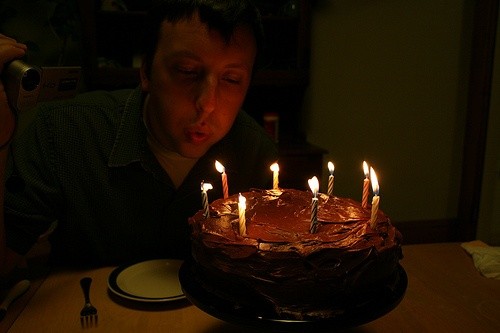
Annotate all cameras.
[1,57,82,112]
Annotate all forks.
[79,277,98,328]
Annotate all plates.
[106,258,187,302]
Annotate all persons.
[0,0,278,278]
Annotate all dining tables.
[0,240,499,331]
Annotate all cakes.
[178,188,402,321]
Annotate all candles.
[270,163,280,189]
[214,161,229,198]
[327,163,335,195]
[370,166,381,227]
[362,161,371,208]
[200,179,213,217]
[237,192,248,238]
[308,176,321,233]
